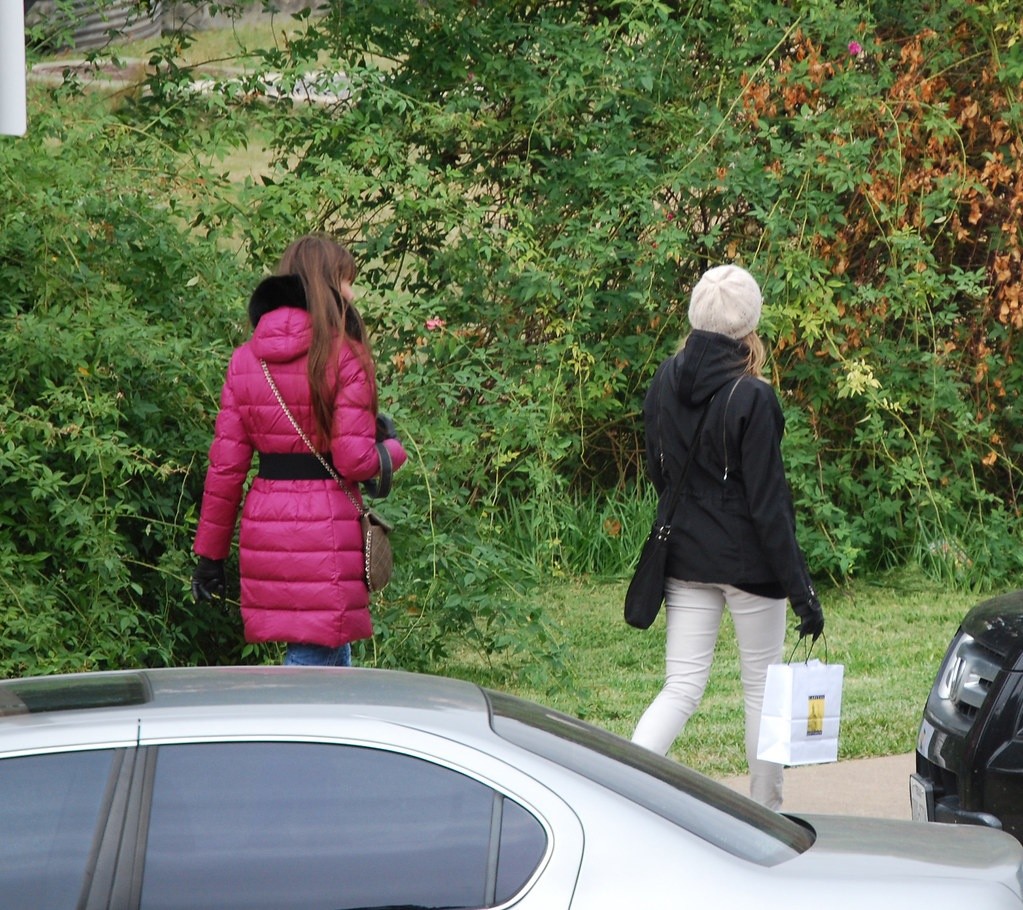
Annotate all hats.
[688,265,762,340]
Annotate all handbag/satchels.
[360,511,394,591]
[757,630,844,766]
[624,520,673,630]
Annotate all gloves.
[376,415,397,442]
[191,557,226,607]
[794,610,825,642]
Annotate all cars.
[906,580,1023,840]
[0,669,1023,910]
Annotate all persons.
[190,234,407,666]
[628,264,825,813]
